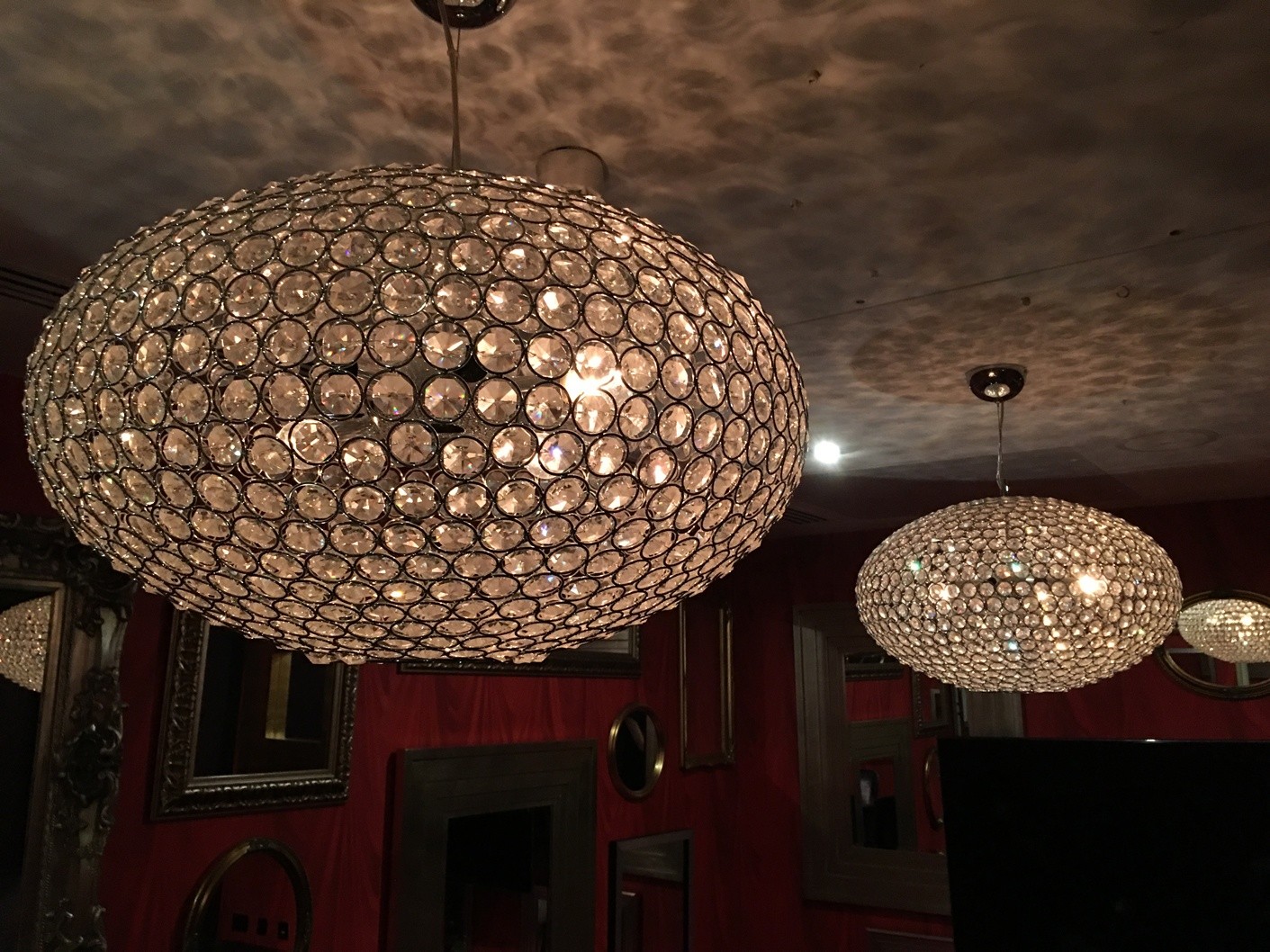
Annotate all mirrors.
[925,749,944,826]
[144,600,359,820]
[0,509,138,952]
[609,702,664,798]
[790,603,1024,913]
[1153,590,1270,698]
[398,625,640,681]
[845,715,917,852]
[912,668,950,736]
[387,739,597,952]
[679,601,733,769]
[607,829,690,952]
[183,835,312,952]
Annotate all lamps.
[23,0,811,664]
[1178,600,1270,663]
[856,361,1182,692]
[1,596,54,693]
